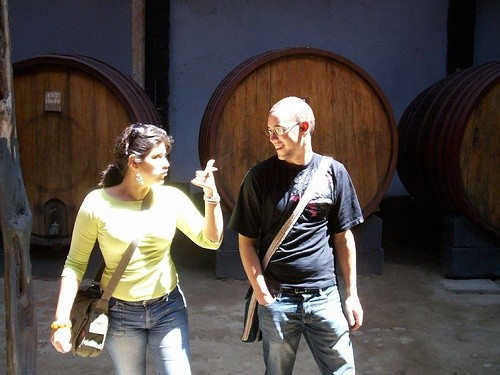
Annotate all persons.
[51,123,224,375]
[227,96,366,375]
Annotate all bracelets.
[51,321,72,330]
[203,195,220,205]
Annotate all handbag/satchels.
[67,289,109,358]
[239,284,265,343]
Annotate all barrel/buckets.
[0,52,162,246]
[198,47,398,229]
[396,62,500,230]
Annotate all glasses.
[265,122,300,136]
[128,123,147,148]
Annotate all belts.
[271,285,337,295]
